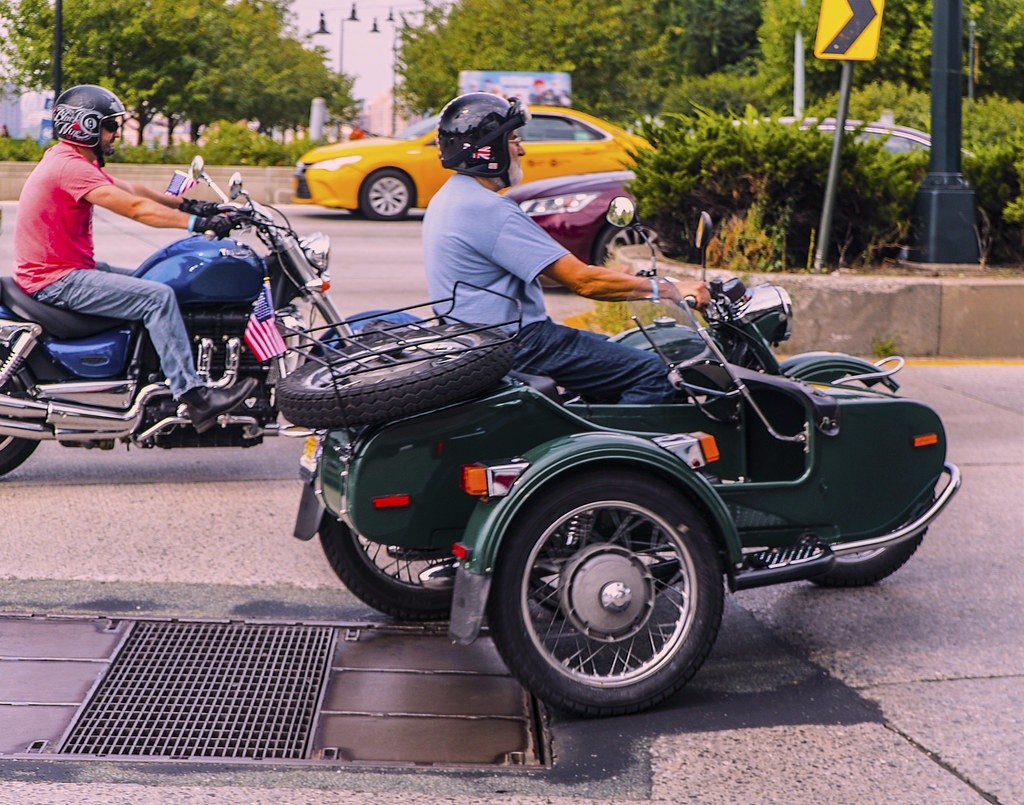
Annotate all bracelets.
[650,280,658,301]
[187,214,197,232]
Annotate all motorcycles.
[265,197,968,714]
[1,154,439,484]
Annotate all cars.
[285,70,658,219]
[500,117,986,256]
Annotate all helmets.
[52,86,128,148]
[437,92,532,176]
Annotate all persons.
[471,79,502,96]
[14,84,258,431]
[529,80,569,105]
[424,93,711,400]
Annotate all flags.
[166,172,199,196]
[244,284,288,362]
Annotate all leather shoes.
[181,376,258,434]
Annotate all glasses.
[505,137,522,144]
[100,119,124,133]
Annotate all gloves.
[186,214,232,241]
[180,197,216,217]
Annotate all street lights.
[304,0,408,136]
